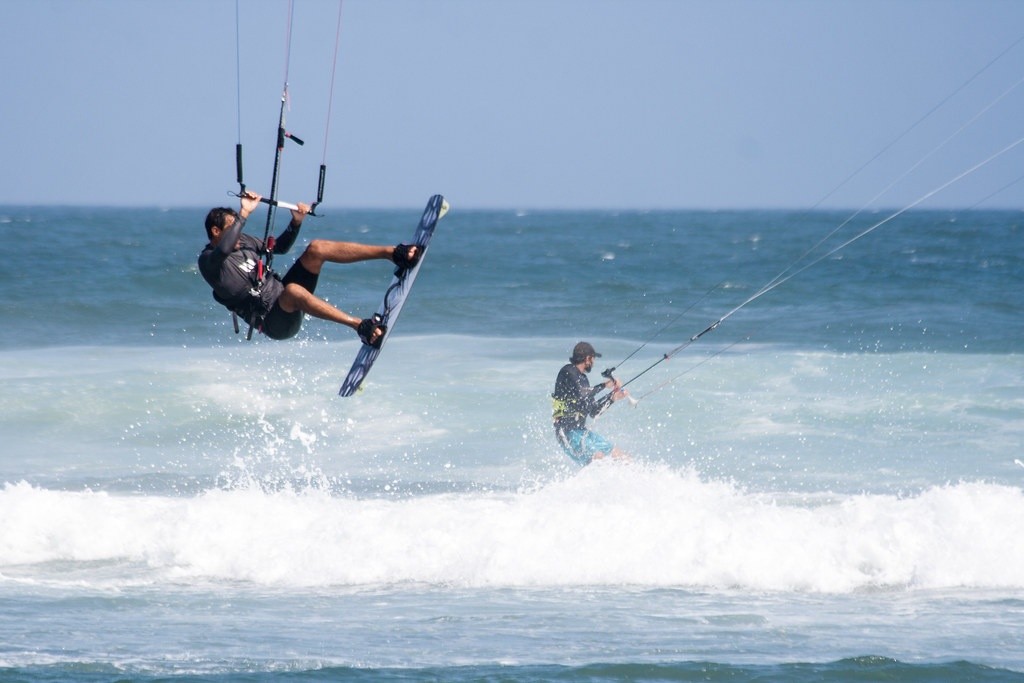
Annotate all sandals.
[357,318,386,349]
[393,244,423,278]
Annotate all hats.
[573,341,602,358]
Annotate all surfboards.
[337,193,445,400]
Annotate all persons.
[553,342,626,467]
[199,192,421,348]
